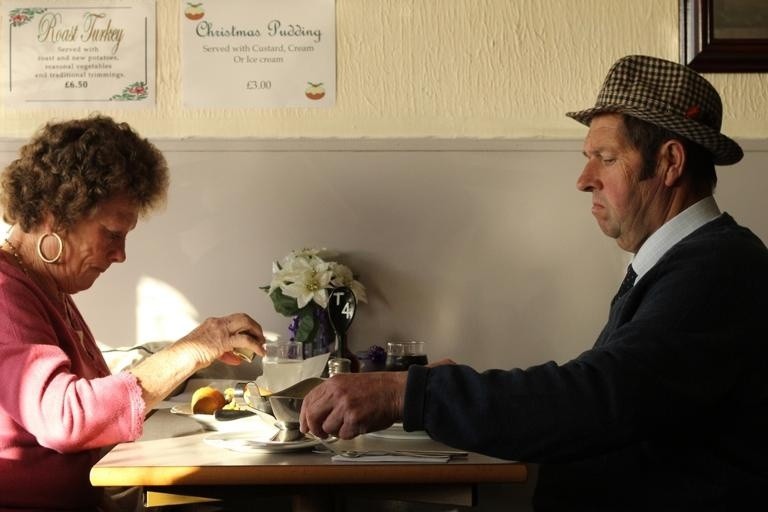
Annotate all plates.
[247,437,337,453]
[169,404,217,431]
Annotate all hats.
[565,55,743,165]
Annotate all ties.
[611,263,637,306]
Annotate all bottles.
[328,358,352,378]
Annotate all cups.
[384,341,427,371]
[263,341,303,362]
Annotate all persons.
[298,53,768,512]
[0,116,268,512]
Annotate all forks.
[319,438,449,459]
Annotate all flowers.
[260,246,371,358]
